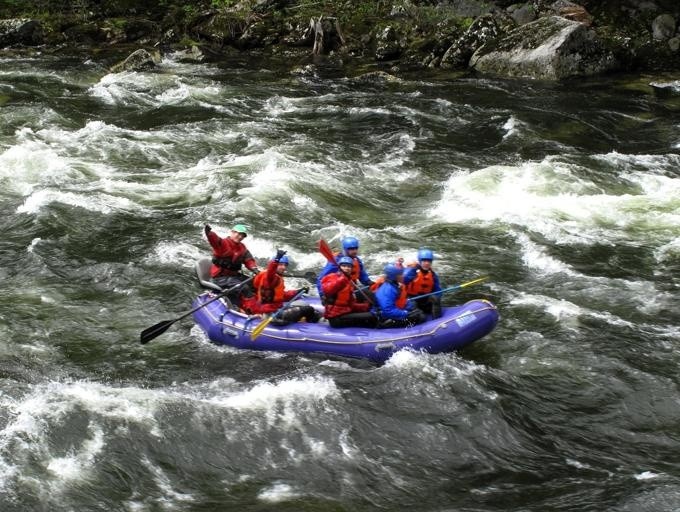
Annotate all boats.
[192,253,499,367]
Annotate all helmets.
[337,257,353,269]
[272,256,289,264]
[343,238,358,251]
[418,249,433,262]
[231,224,247,238]
[385,264,402,281]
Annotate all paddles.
[282,271,318,284]
[407,276,489,301]
[250,286,309,341]
[141,276,254,345]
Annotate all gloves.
[276,248,287,261]
[252,268,260,274]
[204,225,210,232]
[298,286,308,294]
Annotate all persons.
[255,247,319,323]
[321,257,377,328]
[404,249,442,319]
[376,263,425,328]
[205,224,260,302]
